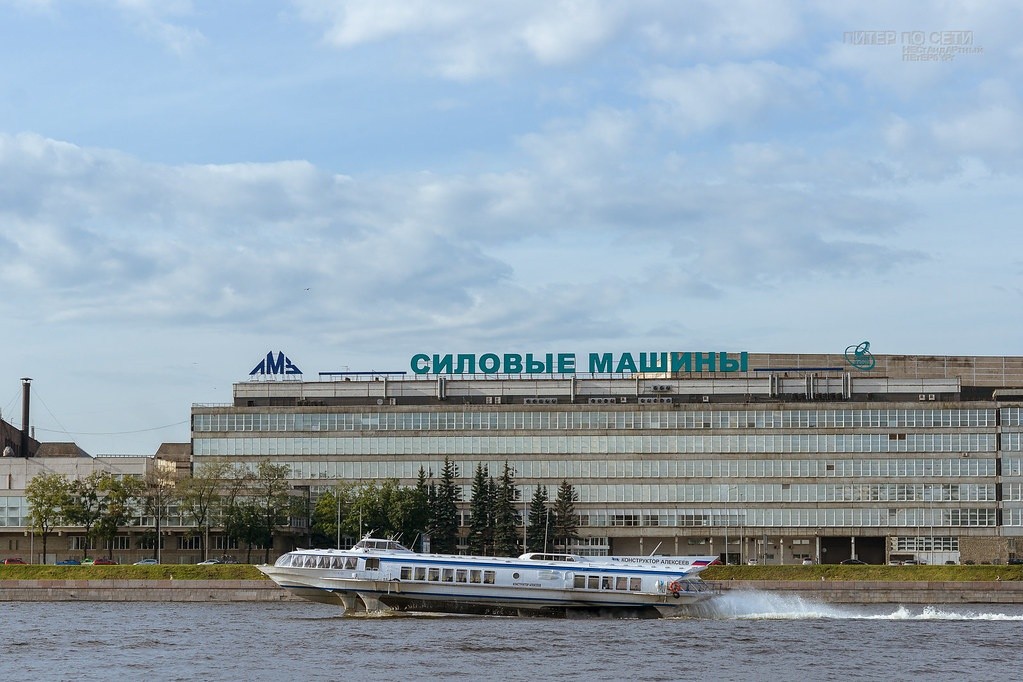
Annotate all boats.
[253,493,725,621]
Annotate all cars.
[747,558,759,566]
[92,558,118,565]
[0,557,32,565]
[53,559,82,565]
[1006,559,1023,565]
[963,559,975,566]
[709,559,722,565]
[839,559,869,565]
[133,559,159,565]
[888,560,917,566]
[197,559,219,565]
[802,557,813,566]
[945,561,956,565]
